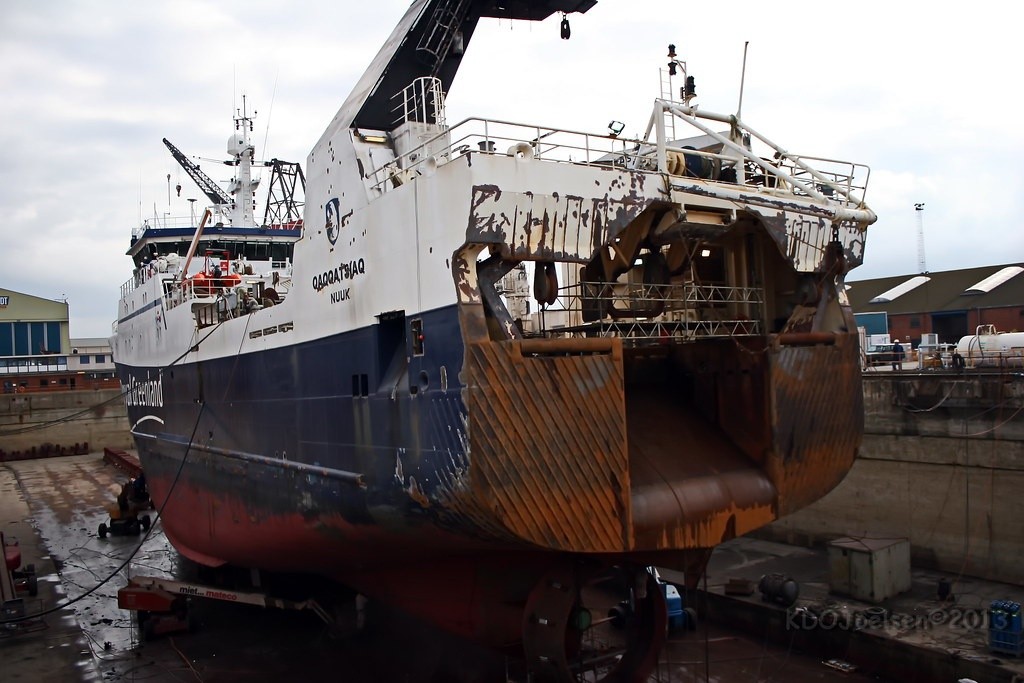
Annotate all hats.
[894,339,899,342]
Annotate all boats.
[106,0,880,683]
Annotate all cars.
[865,345,905,366]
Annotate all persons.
[170,261,276,322]
[891,339,904,371]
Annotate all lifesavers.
[507,143,533,159]
